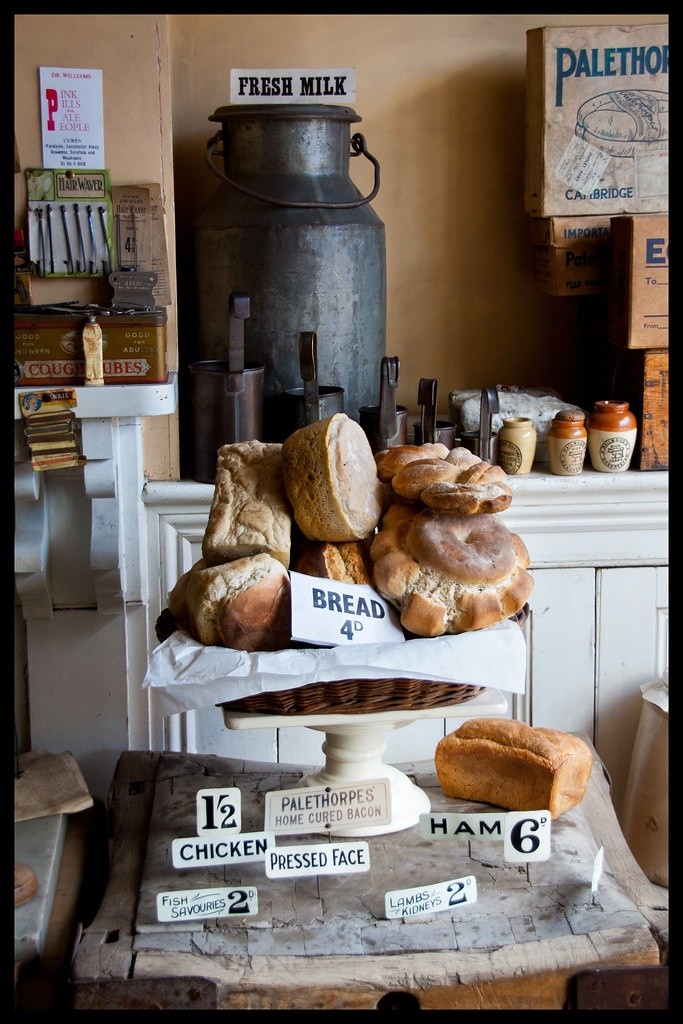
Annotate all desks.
[67,730,668,1010]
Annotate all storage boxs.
[607,220,670,349]
[14,302,166,385]
[526,23,668,218]
[633,349,669,472]
[534,212,667,295]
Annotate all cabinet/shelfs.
[137,462,669,774]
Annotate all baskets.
[156,601,530,715]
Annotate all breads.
[168,412,534,652]
[434,717,592,820]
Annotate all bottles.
[548,418,586,475]
[589,401,637,473]
[497,417,538,475]
